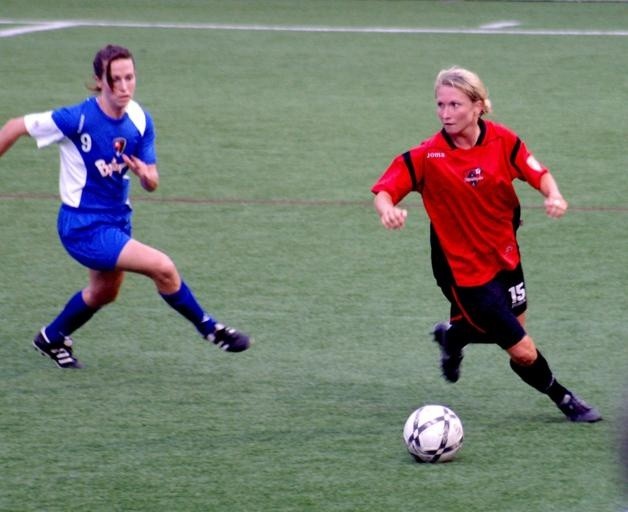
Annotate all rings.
[553,200,560,206]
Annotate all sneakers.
[32,326,83,369]
[197,322,250,353]
[556,391,602,423]
[432,321,464,383]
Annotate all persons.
[368,64,603,425]
[1,44,254,369]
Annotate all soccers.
[402,406,462,460]
